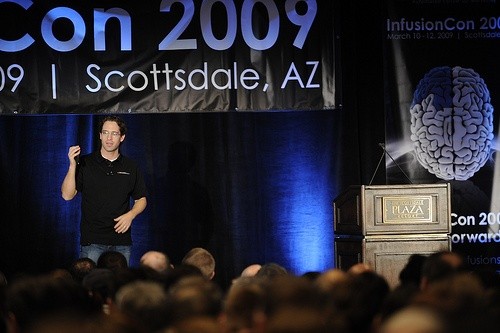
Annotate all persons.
[0,244,500,333]
[61,116,148,270]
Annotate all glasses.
[100,131,121,137]
